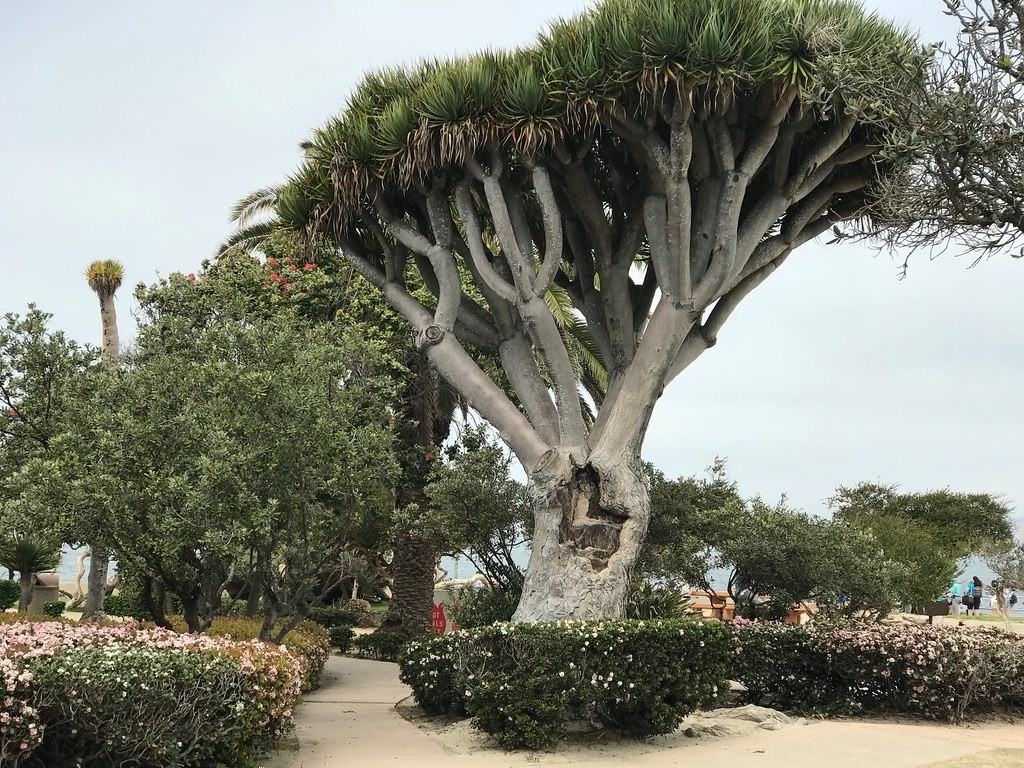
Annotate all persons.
[945,576,1014,618]
[965,576,983,616]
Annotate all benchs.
[692,603,806,624]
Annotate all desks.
[691,590,771,621]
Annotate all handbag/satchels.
[1010,594,1017,606]
[962,594,974,605]
